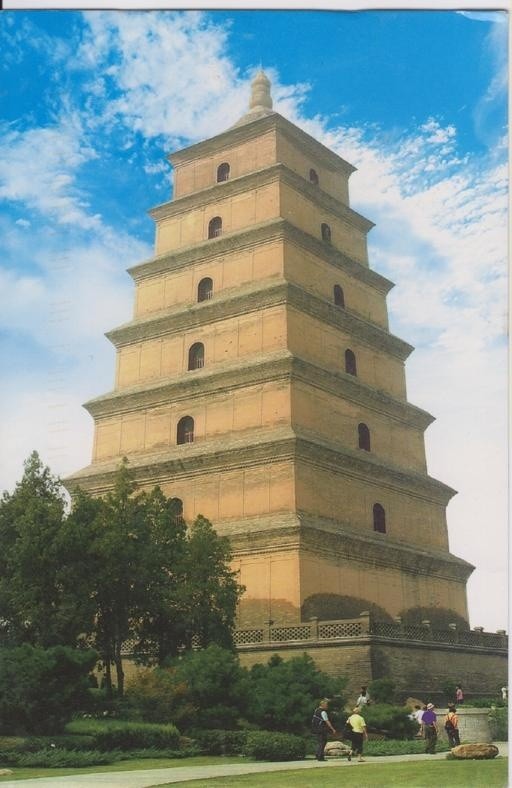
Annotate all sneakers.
[423,747,436,754]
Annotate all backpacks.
[343,722,353,740]
[311,707,322,735]
[444,720,455,735]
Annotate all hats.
[427,703,435,710]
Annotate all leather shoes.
[315,753,366,762]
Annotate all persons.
[443,707,460,748]
[412,704,426,728]
[343,707,368,763]
[355,691,367,711]
[420,702,438,754]
[310,699,336,761]
[447,701,455,710]
[500,685,508,700]
[455,684,464,704]
[359,685,373,704]
